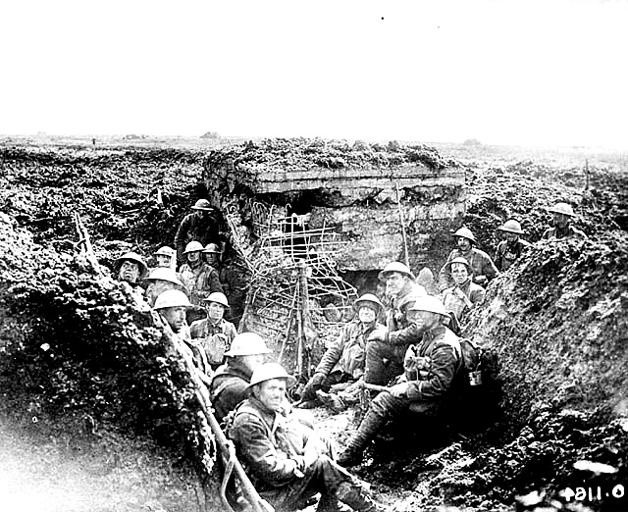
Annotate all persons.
[119,201,591,510]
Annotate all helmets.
[190,199,214,211]
[113,240,296,397]
[444,202,577,276]
[352,262,453,321]
